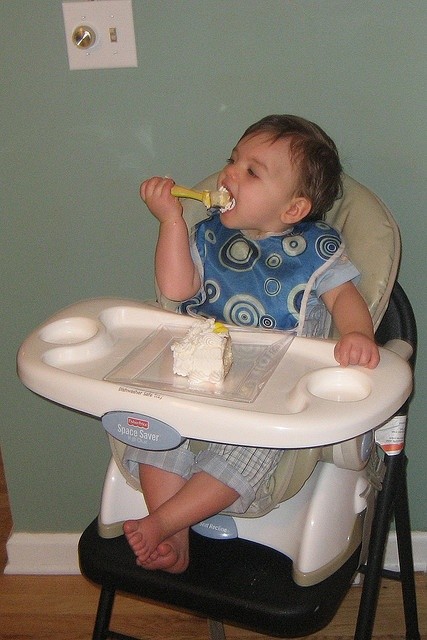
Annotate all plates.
[104,321,296,404]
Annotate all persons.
[124,113,379,574]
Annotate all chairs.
[78,281,418,640]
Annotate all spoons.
[171,183,232,208]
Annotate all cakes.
[170,319,235,386]
[217,186,236,214]
